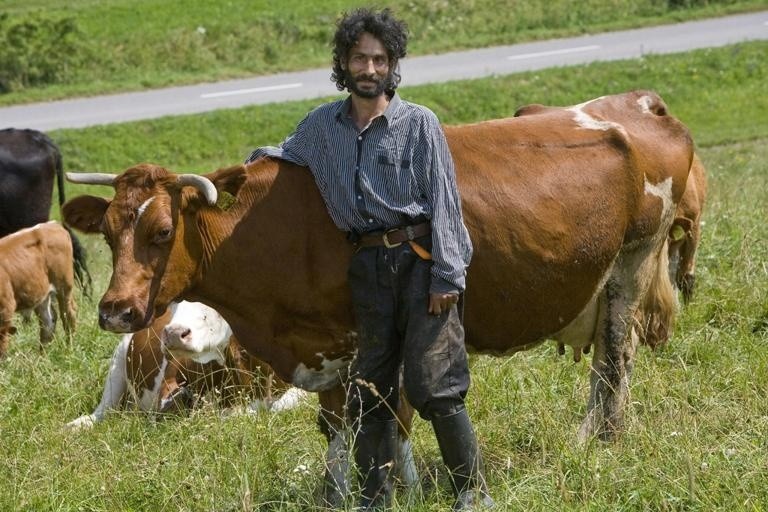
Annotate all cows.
[0,125,92,297]
[62,88,710,458]
[62,301,308,433]
[0,218,96,362]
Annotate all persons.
[249,6,494,512]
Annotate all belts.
[362,222,433,250]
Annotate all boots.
[431,410,493,510]
[353,422,399,512]
